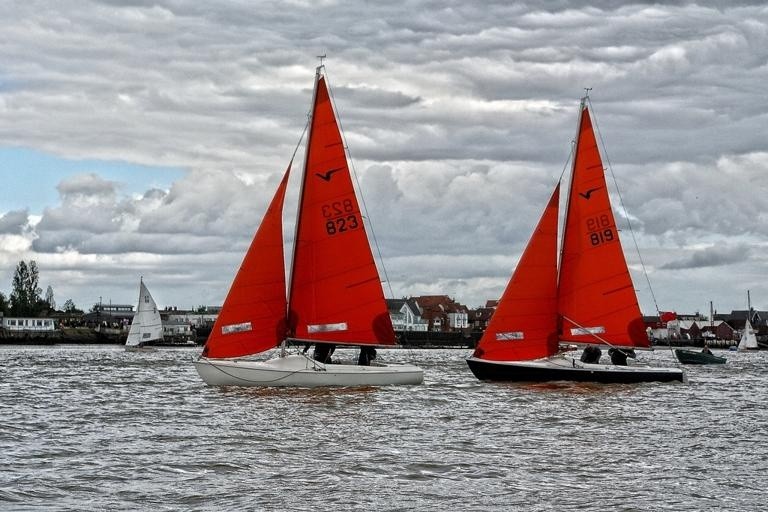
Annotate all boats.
[675,347,727,365]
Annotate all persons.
[581,344,603,364]
[302,341,336,363]
[608,348,635,365]
[357,345,376,365]
[700,345,712,355]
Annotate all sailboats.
[736,320,762,352]
[191,54,429,387]
[467,84,686,390]
[122,274,164,353]
